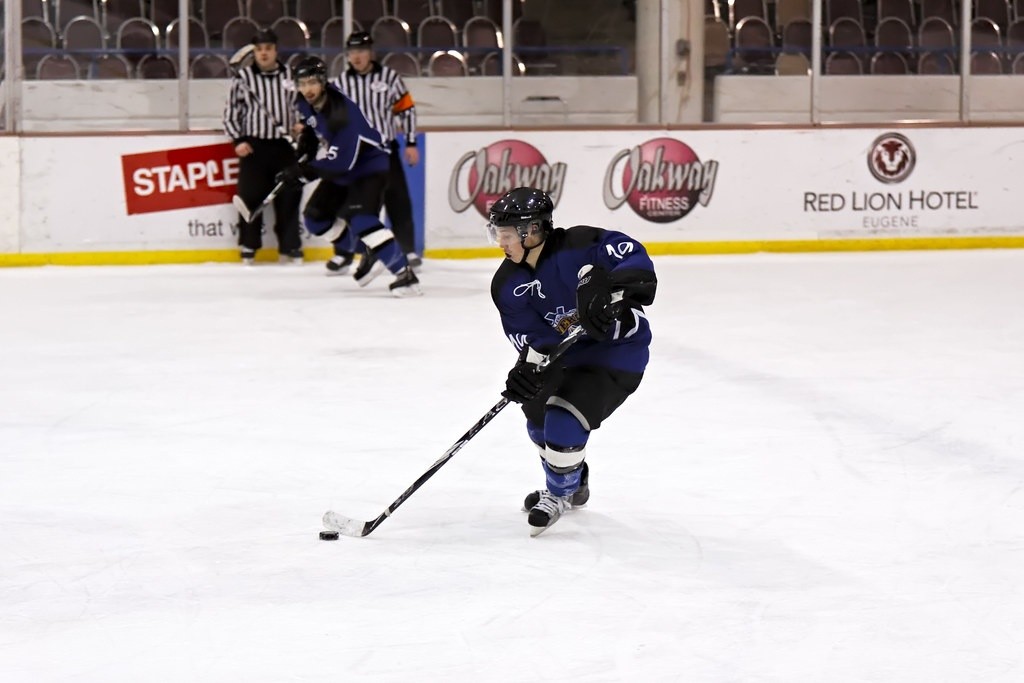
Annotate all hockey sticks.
[228,44,299,149]
[231,152,308,224]
[321,306,622,538]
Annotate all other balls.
[318,530,340,542]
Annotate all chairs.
[704,0,1024,74]
[0,0,549,80]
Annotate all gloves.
[507,362,539,404]
[275,169,302,194]
[577,264,615,341]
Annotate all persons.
[222,28,305,265]
[325,32,421,275]
[276,56,419,297]
[486,187,657,537]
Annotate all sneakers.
[326,254,353,276]
[278,248,304,264]
[524,483,589,537]
[353,253,385,287]
[407,252,422,266]
[241,252,255,264]
[389,266,424,297]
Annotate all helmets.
[293,56,325,77]
[251,29,277,44]
[490,187,554,227]
[347,32,374,48]
